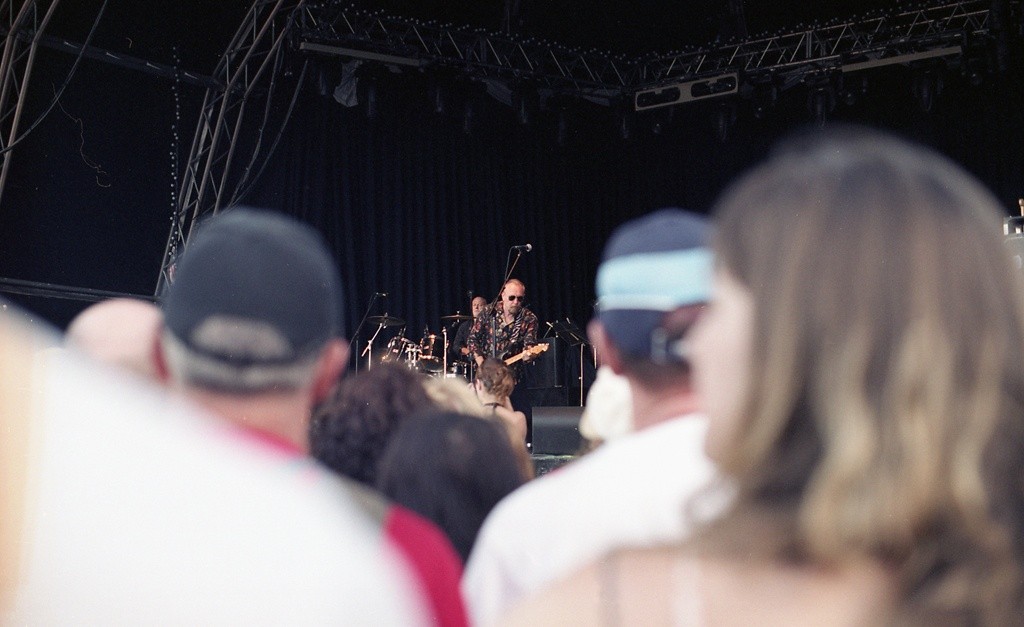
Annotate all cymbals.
[365,316,405,327]
[441,314,476,319]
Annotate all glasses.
[503,293,525,302]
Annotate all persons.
[458,138,1024,627]
[0,210,535,625]
[452,279,539,454]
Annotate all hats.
[594,209,723,311]
[160,207,344,366]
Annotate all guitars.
[497,343,550,366]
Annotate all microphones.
[513,244,533,252]
[401,327,407,337]
[426,324,430,336]
[375,293,387,296]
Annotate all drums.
[418,335,450,372]
[382,336,420,373]
[447,360,471,380]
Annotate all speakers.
[522,337,590,455]
